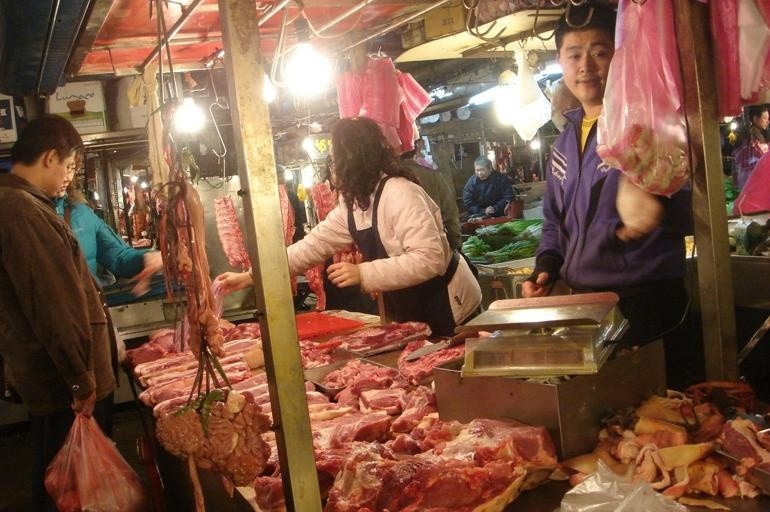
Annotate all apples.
[44,467,142,512]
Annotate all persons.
[51,144,163,300]
[735,103,769,150]
[0,113,118,512]
[462,155,516,226]
[211,115,486,341]
[520,3,692,392]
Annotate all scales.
[451,290,631,380]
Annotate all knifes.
[404,330,477,363]
